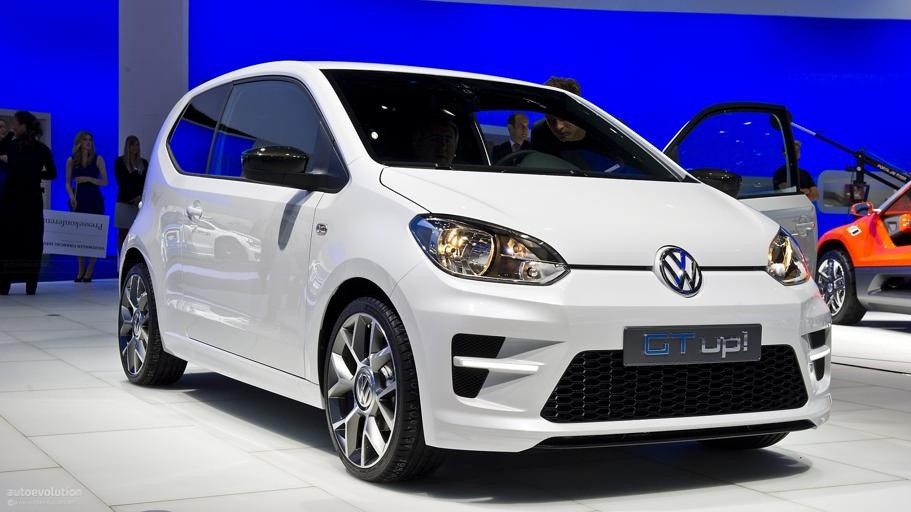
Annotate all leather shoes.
[73,268,93,282]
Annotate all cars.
[812,176,911,330]
[113,57,838,484]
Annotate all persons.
[490,111,532,167]
[64,128,108,282]
[424,120,459,164]
[0,118,8,193]
[531,74,634,176]
[113,133,148,255]
[0,109,58,295]
[772,139,820,202]
